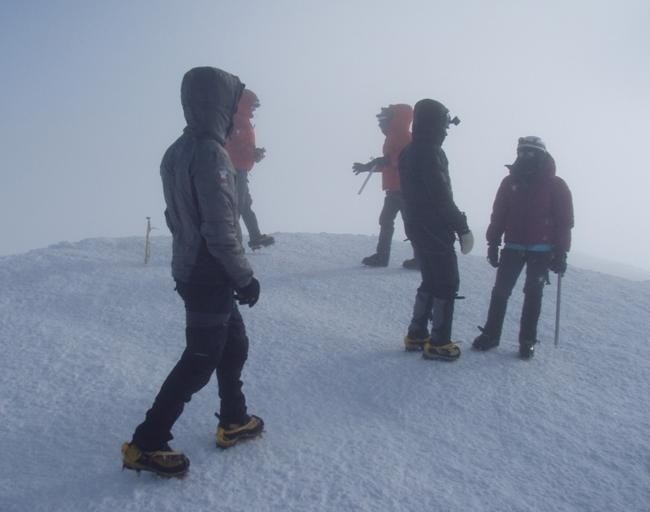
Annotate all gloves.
[488,244,500,268]
[233,276,260,307]
[547,251,567,270]
[461,231,473,255]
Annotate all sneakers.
[424,341,460,361]
[403,258,419,269]
[215,414,263,447]
[403,335,429,350]
[361,254,389,267]
[518,341,535,358]
[120,440,188,475]
[472,333,498,349]
[247,234,274,249]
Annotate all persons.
[223,88,276,251]
[349,100,421,269]
[468,133,578,363]
[120,65,264,478]
[393,97,474,360]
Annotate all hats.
[375,111,388,122]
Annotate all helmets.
[518,136,545,160]
